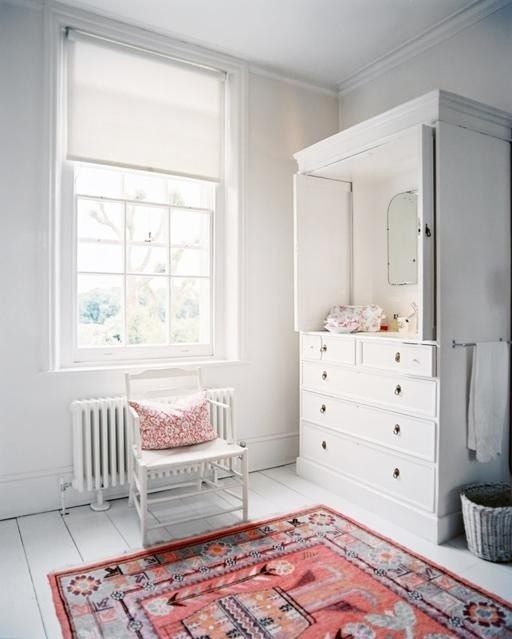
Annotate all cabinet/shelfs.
[292,90,512,546]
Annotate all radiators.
[59,386,239,517]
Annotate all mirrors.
[386,190,419,286]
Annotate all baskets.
[459,479,512,562]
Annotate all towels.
[465,341,510,465]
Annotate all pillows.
[128,397,219,450]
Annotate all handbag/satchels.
[323,304,385,332]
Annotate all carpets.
[46,502,511,639]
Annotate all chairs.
[124,365,250,547]
